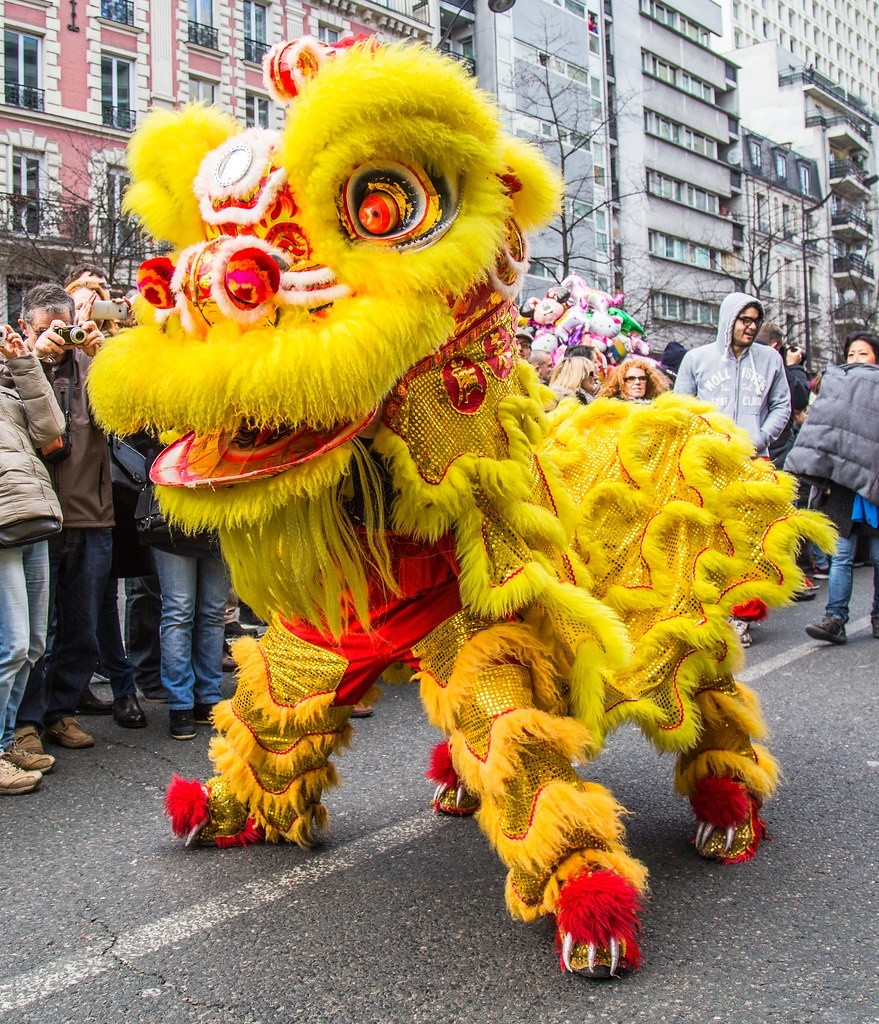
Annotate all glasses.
[516,341,530,348]
[737,316,763,328]
[99,283,112,291]
[29,323,47,339]
[624,376,648,382]
[586,371,593,376]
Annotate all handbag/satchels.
[38,432,71,463]
[135,480,182,545]
[106,434,148,493]
[0,517,62,549]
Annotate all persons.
[782,332,879,643]
[0,263,269,796]
[515,327,688,404]
[661,292,831,649]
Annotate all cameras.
[780,346,806,365]
[53,326,87,346]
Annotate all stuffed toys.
[84,34,839,978]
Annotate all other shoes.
[222,599,270,670]
[141,686,169,703]
[352,700,374,717]
[791,567,829,602]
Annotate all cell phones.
[93,301,127,320]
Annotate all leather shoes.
[193,703,218,724]
[112,693,147,728]
[169,709,196,739]
[74,687,114,715]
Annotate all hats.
[662,341,688,368]
[516,327,534,342]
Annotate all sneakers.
[728,617,752,647]
[44,716,95,748]
[871,616,879,638]
[15,725,44,754]
[2,740,55,773]
[805,614,847,644]
[0,752,42,794]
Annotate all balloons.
[516,271,650,375]
